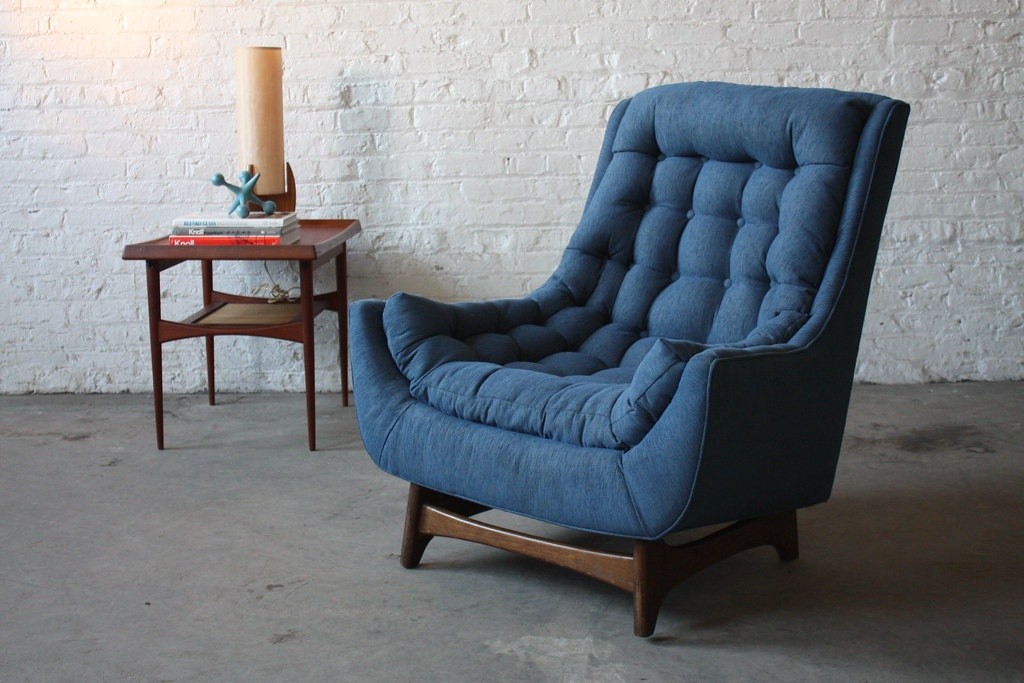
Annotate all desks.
[122,219,362,451]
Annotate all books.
[169,212,301,246]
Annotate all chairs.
[347,82,910,638]
